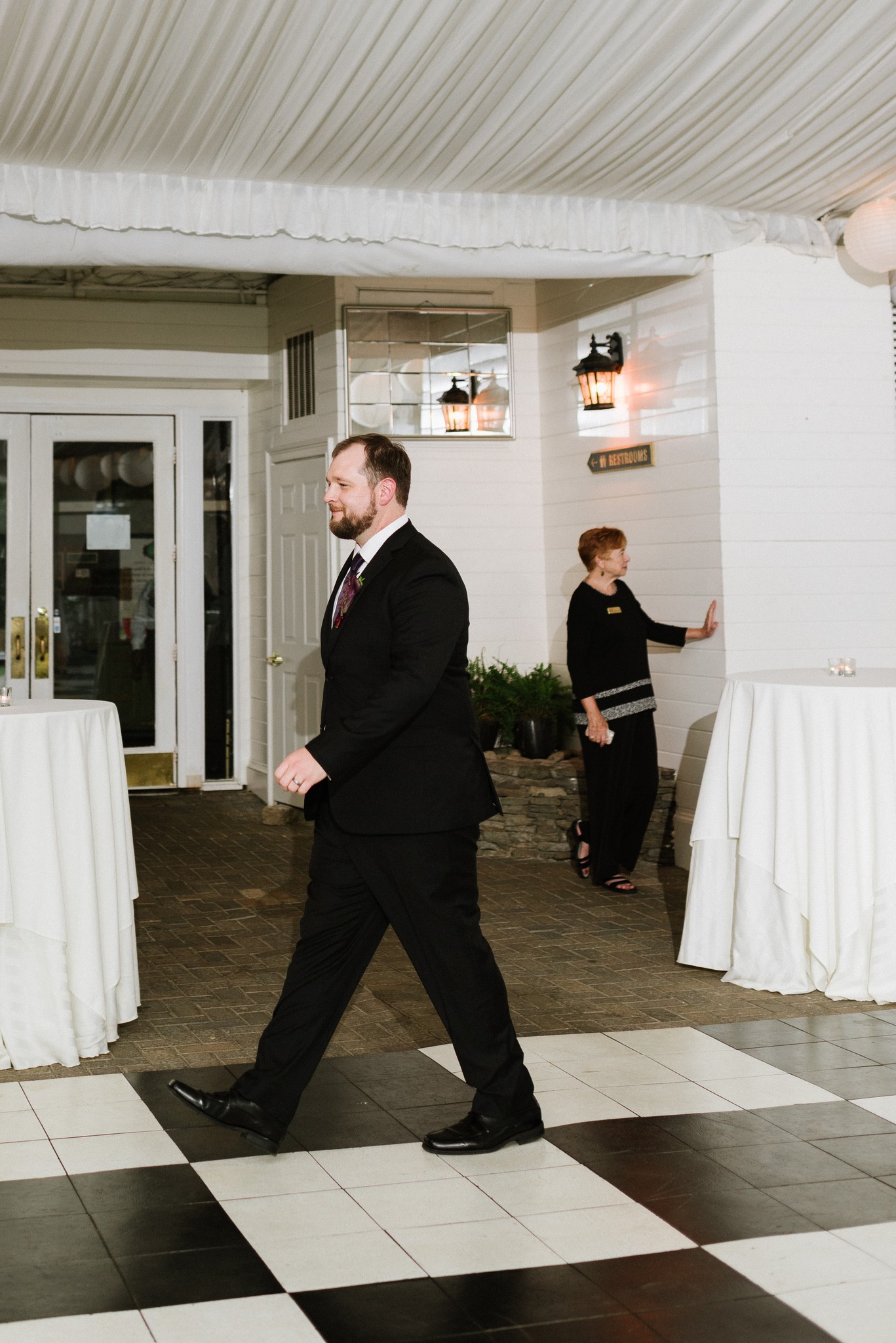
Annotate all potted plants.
[468,647,575,759]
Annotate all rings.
[292,778,301,786]
[590,736,595,739]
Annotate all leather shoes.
[169,1079,289,1156]
[421,1096,545,1156]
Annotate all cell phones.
[585,726,614,744]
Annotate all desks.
[677,666,896,1007]
[1,698,141,1072]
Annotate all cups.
[828,658,839,675]
[839,658,856,676]
[0,687,12,707]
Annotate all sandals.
[572,818,591,878]
[594,873,637,893]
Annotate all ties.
[332,553,365,627]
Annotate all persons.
[166,433,546,1155]
[567,527,717,892]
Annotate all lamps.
[437,375,488,431]
[474,368,511,433]
[572,329,624,412]
[843,197,896,274]
